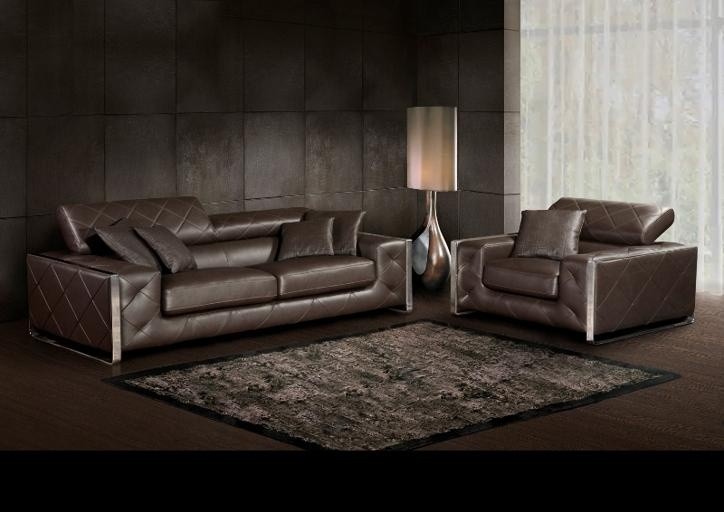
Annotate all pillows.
[510,210,585,263]
[93,220,157,272]
[133,222,196,270]
[275,220,332,260]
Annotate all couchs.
[27,196,412,367]
[450,195,697,345]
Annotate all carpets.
[99,318,681,450]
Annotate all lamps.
[407,106,457,287]
